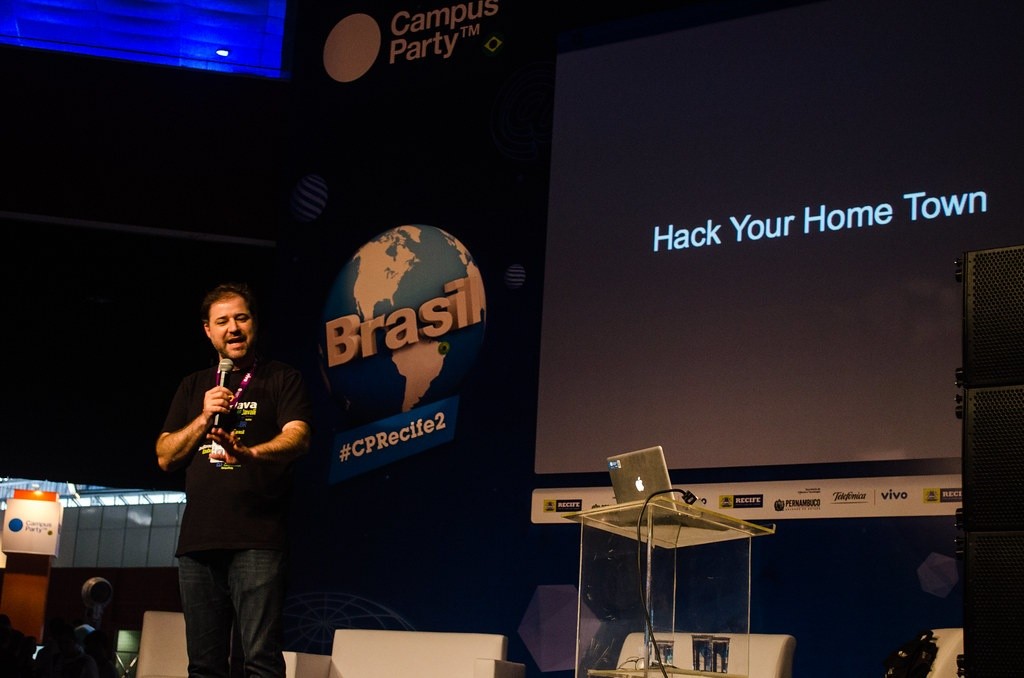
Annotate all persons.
[0,615,118,678]
[153,284,309,678]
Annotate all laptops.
[608,445,729,532]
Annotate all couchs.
[282,628,525,678]
[614,632,798,678]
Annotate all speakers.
[956,244,1024,678]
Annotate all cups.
[692,634,730,673]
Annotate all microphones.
[214,358,233,428]
